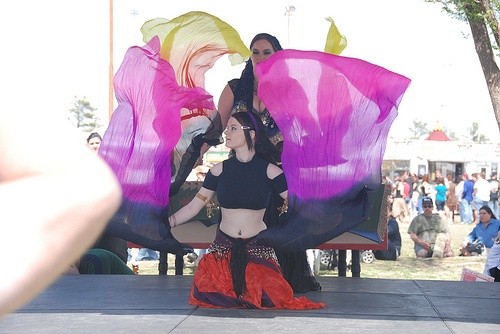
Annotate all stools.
[448,204,460,222]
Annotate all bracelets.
[168,214,178,228]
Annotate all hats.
[422,198,433,205]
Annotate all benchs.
[157,181,392,277]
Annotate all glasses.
[423,205,433,208]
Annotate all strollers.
[319,249,374,269]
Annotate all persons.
[172,33,322,295]
[168,110,325,308]
[87,131,128,264]
[126,157,500,282]
[63,247,135,276]
[0,82,123,319]
